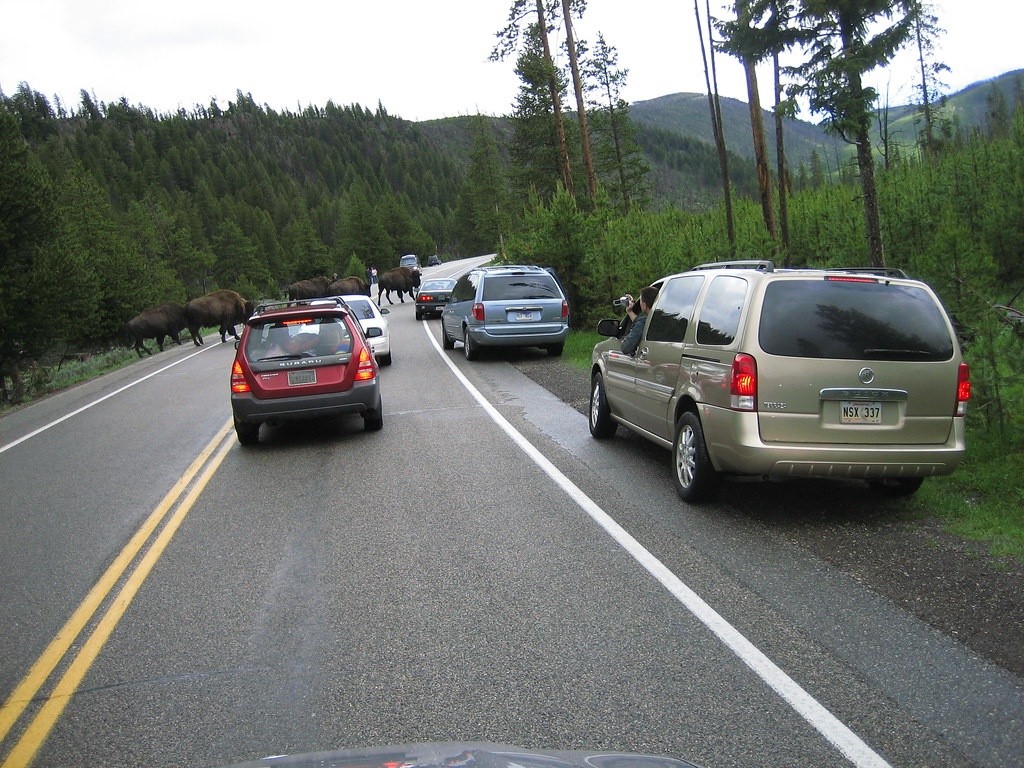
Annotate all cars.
[413,278,458,319]
[297,295,392,367]
[427,255,441,267]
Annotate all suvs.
[586,259,969,503]
[439,265,570,360]
[230,297,383,445]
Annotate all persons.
[332,272,339,281]
[263,322,292,344]
[620,287,658,354]
[367,265,379,284]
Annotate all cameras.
[613,296,631,307]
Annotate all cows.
[327,275,371,297]
[377,266,422,308]
[181,289,256,346]
[284,273,337,308]
[125,302,189,358]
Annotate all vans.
[399,254,423,277]
